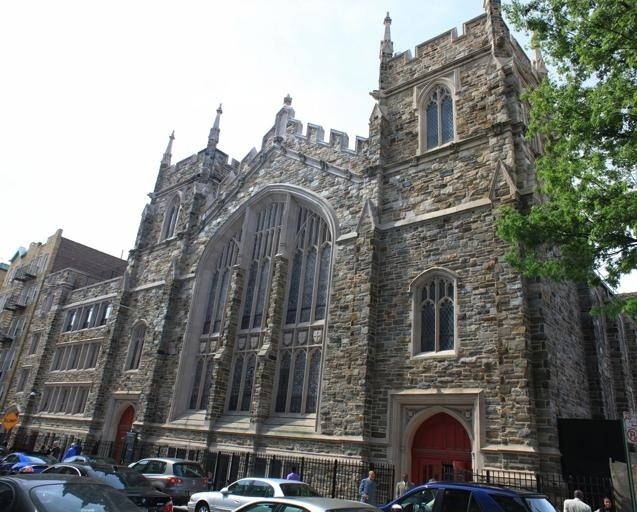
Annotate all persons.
[358,470,376,505]
[421,478,439,504]
[49,440,59,458]
[62,442,76,458]
[73,441,81,455]
[286,466,300,480]
[594,495,613,511]
[394,472,415,499]
[563,490,590,511]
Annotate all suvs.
[379,479,563,512]
[131,457,210,505]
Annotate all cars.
[63,455,119,464]
[230,495,384,512]
[187,477,323,512]
[39,462,173,512]
[0,474,143,512]
[0,452,60,475]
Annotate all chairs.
[151,464,161,474]
